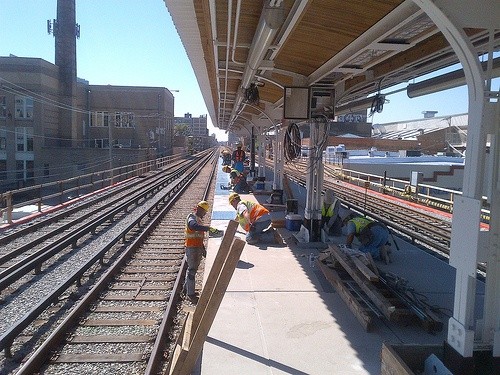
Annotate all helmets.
[237,143,243,148]
[197,201,210,212]
[222,165,227,173]
[228,193,240,205]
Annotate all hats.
[324,189,334,205]
[339,209,354,220]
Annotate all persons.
[321,190,343,234]
[228,193,283,245]
[342,216,392,265]
[184,200,220,304]
[222,138,249,194]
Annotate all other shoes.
[186,295,199,305]
[183,289,199,296]
[380,245,393,266]
[269,227,283,246]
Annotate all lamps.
[335,91,385,116]
[407,55,500,98]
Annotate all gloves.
[209,227,220,234]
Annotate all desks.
[243,162,263,177]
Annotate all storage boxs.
[254,182,265,191]
[285,212,302,231]
